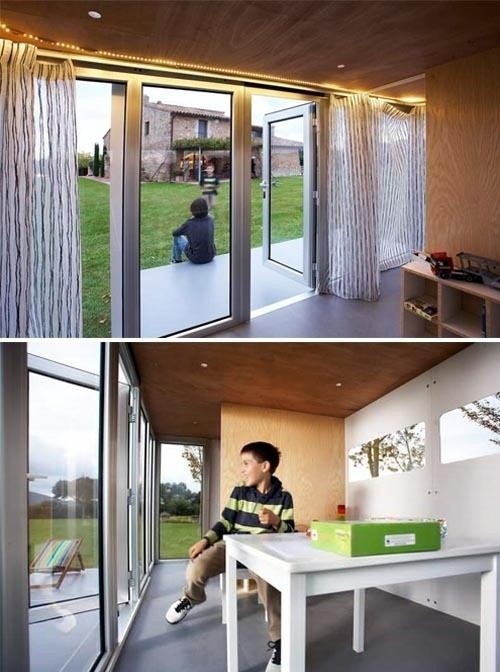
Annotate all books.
[403,293,439,322]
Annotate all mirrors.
[346,419,426,484]
[439,393,500,465]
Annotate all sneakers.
[165,594,195,625]
[266,641,282,672]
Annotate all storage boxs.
[307,518,446,560]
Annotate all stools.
[219,568,274,631]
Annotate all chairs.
[31,533,91,588]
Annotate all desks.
[216,519,499,672]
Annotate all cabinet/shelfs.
[397,254,499,338]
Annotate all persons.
[199,162,220,219]
[163,439,296,672]
[169,197,217,267]
